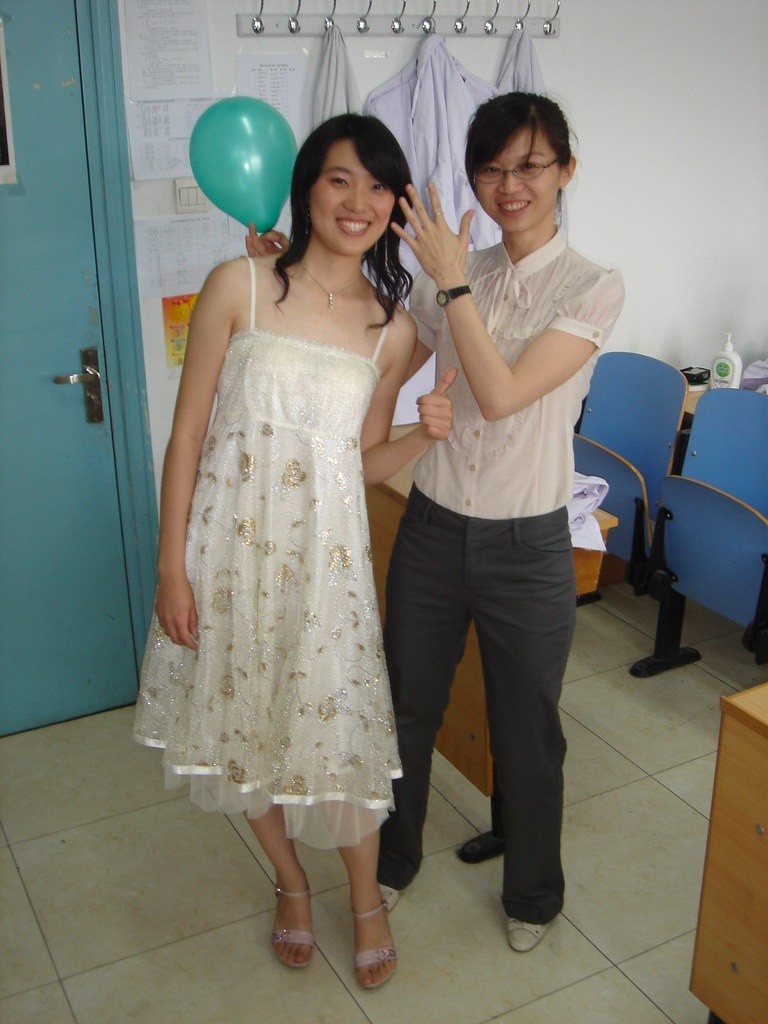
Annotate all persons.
[134,115,456,987]
[243,91,627,954]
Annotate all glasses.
[469,157,560,183]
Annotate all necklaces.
[298,259,363,308]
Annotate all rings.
[435,212,444,216]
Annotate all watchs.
[437,284,474,305]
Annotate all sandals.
[271,884,316,968]
[350,891,398,988]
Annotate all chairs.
[571,351,768,678]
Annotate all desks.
[687,682,768,1024]
[364,422,619,795]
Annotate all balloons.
[191,97,300,233]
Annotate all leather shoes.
[508,918,550,952]
[378,883,401,912]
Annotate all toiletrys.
[710,330,743,390]
